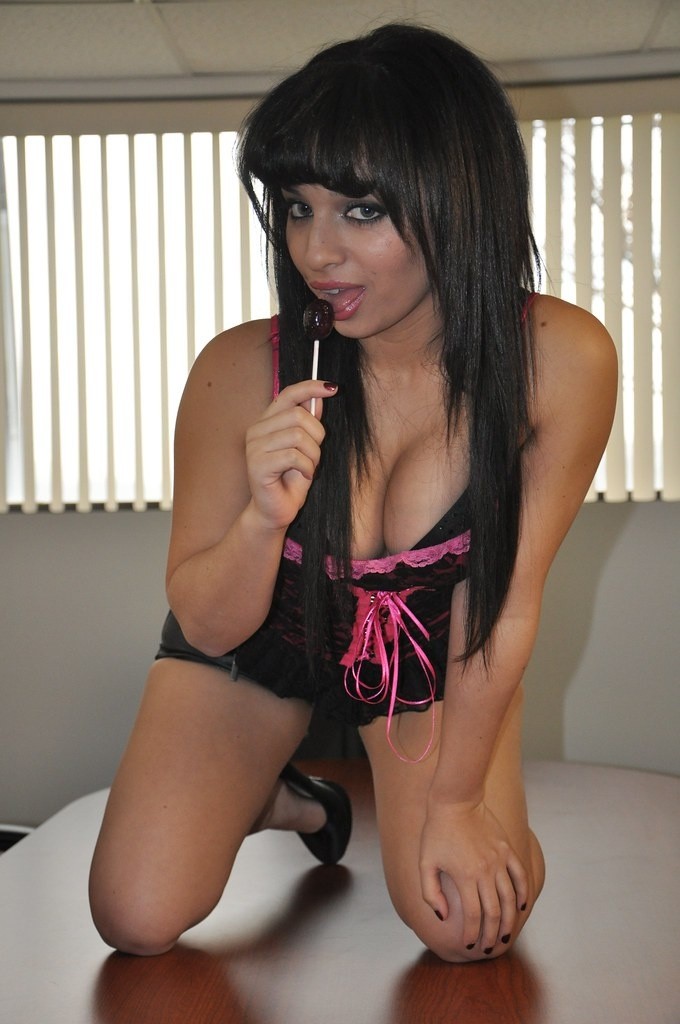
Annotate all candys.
[303,298,334,340]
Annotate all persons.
[89,24,619,963]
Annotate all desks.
[0,759,680,1023]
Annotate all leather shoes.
[275,761,355,868]
